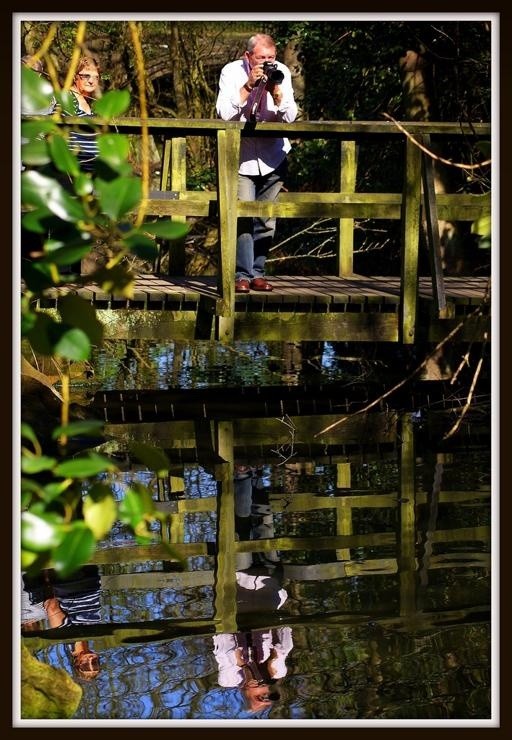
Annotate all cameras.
[257,61,285,88]
[257,691,281,702]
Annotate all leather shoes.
[235,279,250,292]
[251,278,273,290]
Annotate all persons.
[21,54,54,171]
[53,58,109,290]
[214,33,298,292]
[213,459,295,713]
[21,573,50,635]
[24,501,111,681]
